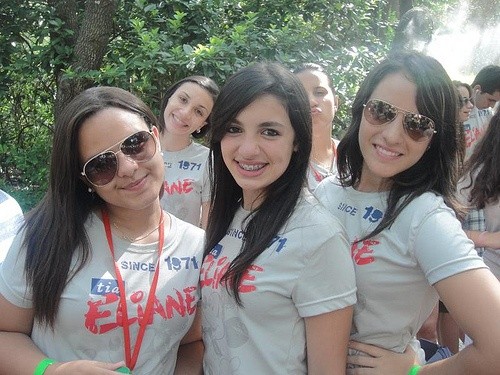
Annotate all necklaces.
[109,211,166,243]
[99,204,164,375]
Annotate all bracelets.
[407,365,422,375]
[32,356,56,375]
[478,230,488,249]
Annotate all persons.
[154,75,217,233]
[0,85,208,375]
[290,62,345,194]
[313,48,499,375]
[415,63,500,375]
[198,62,358,375]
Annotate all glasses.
[79,127,159,187]
[362,98,438,144]
[463,96,475,106]
